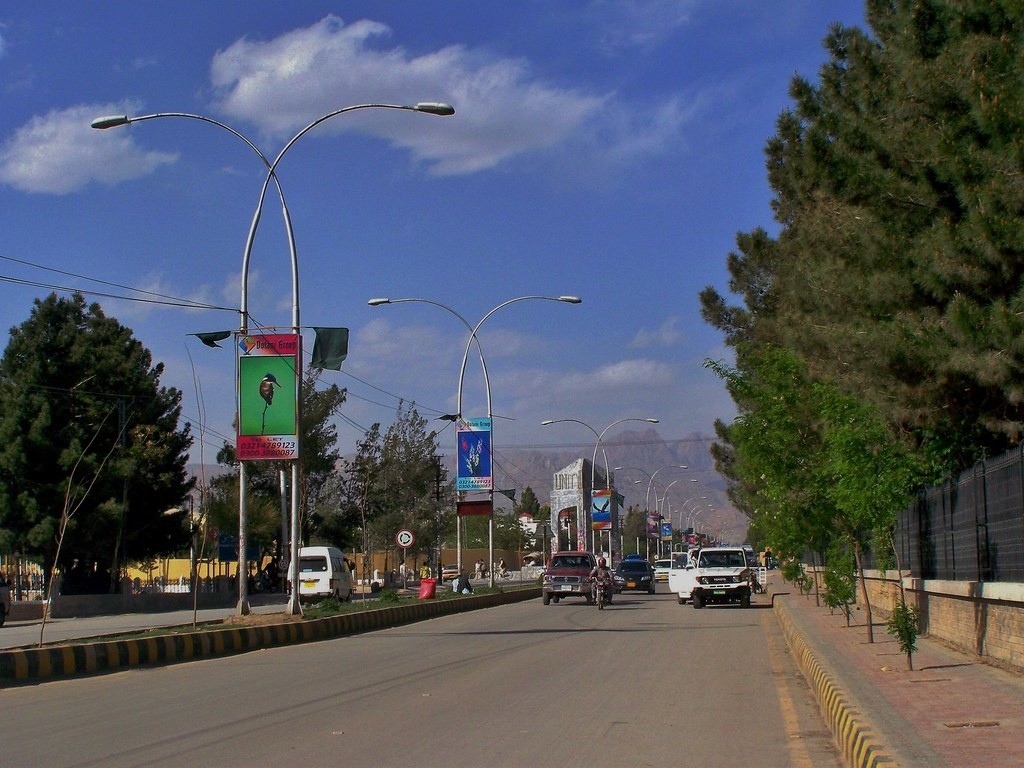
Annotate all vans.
[668,544,760,609]
[285,546,356,605]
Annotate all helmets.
[598,557,606,568]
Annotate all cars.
[651,558,682,583]
[441,563,464,582]
[612,553,656,595]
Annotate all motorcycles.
[586,572,616,611]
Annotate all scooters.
[747,566,763,595]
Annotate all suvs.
[540,550,597,606]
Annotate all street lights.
[540,417,660,569]
[88,102,456,615]
[615,464,715,568]
[367,295,583,594]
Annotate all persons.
[475,557,508,581]
[262,557,280,594]
[764,548,773,570]
[589,557,614,606]
[420,562,431,579]
